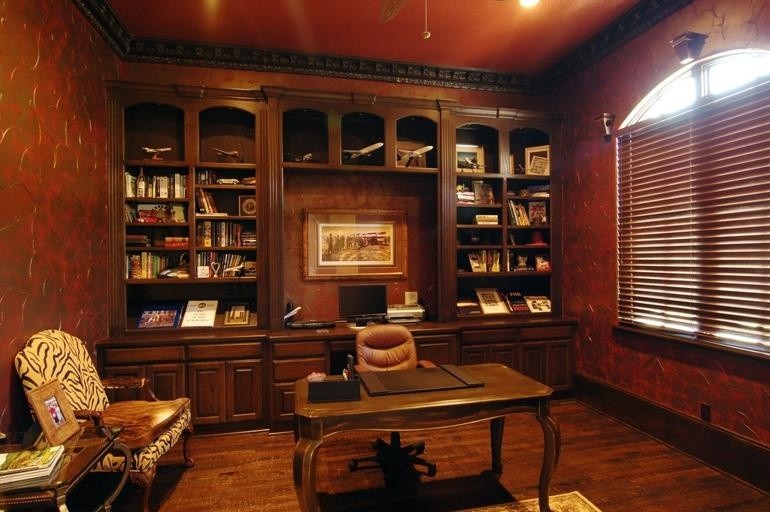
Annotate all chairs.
[347,324,436,477]
[14,330,193,512]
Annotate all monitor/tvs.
[339,284,389,328]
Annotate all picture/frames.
[180,300,219,328]
[502,291,528,312]
[301,206,409,281]
[24,378,80,447]
[223,302,251,325]
[474,287,511,313]
[137,308,183,327]
[523,296,552,313]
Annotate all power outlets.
[700,403,711,422]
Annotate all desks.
[1,425,125,512]
[293,363,562,512]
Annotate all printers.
[388,293,425,323]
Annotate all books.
[0,444,65,492]
[456,180,553,316]
[510,154,514,174]
[124,166,257,279]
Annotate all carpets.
[453,491,603,512]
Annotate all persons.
[47,402,64,426]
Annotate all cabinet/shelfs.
[93,80,579,431]
